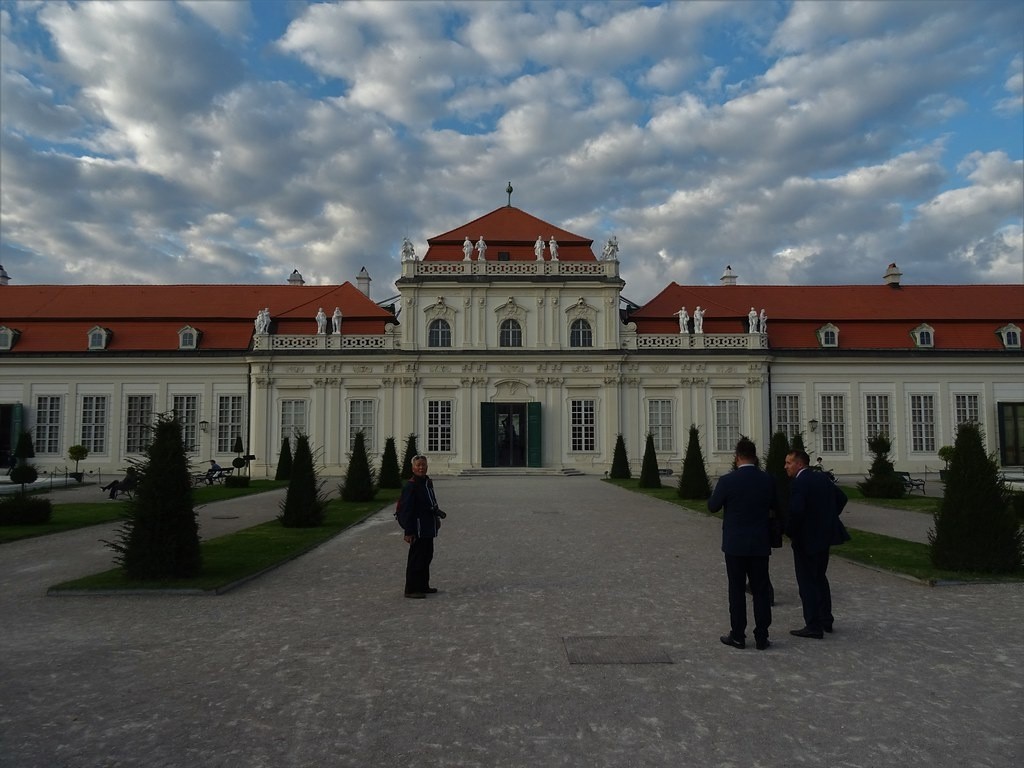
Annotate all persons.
[760,309,768,334]
[402,237,415,259]
[549,236,559,259]
[255,307,272,334]
[673,307,690,332]
[315,308,327,335]
[814,457,840,484]
[706,438,782,650]
[602,235,620,259]
[206,459,222,486]
[783,449,852,639]
[748,307,758,333]
[99,466,137,501]
[534,236,545,260]
[398,455,446,598]
[462,237,474,261]
[694,306,705,332]
[474,236,488,261]
[332,307,343,335]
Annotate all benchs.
[896,471,925,495]
[197,466,234,485]
[115,476,140,500]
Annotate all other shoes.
[720,636,746,649]
[404,593,427,598]
[790,626,825,639]
[425,587,437,593]
[755,639,770,650]
[824,624,833,633]
